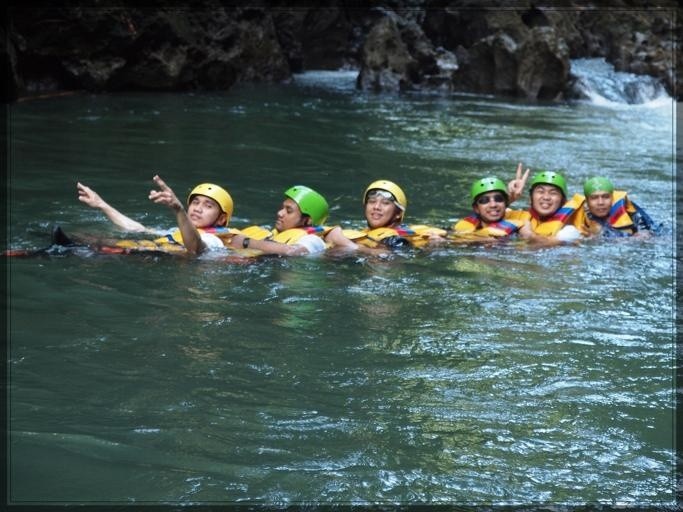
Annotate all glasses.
[478,194,506,204]
[367,189,406,212]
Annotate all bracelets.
[243,237,250,248]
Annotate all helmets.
[528,170,568,201]
[469,177,510,209]
[361,178,408,224]
[186,181,234,227]
[283,184,330,228]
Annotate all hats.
[582,175,615,198]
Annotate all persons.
[343,180,448,251]
[454,176,526,249]
[230,185,393,259]
[503,162,590,251]
[580,176,665,240]
[1,174,245,263]
